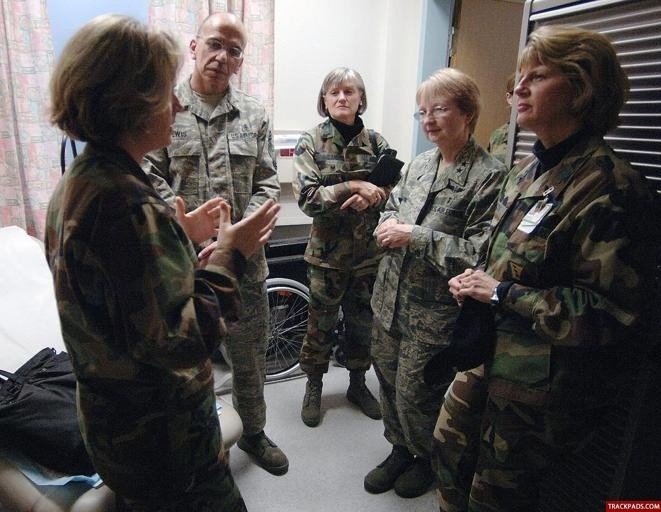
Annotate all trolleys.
[217,181,315,386]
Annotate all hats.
[424,298,494,381]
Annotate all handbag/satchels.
[366,154,404,187]
[0,345,93,475]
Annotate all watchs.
[489,282,503,309]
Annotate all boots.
[365,442,413,496]
[395,456,436,497]
[302,374,324,428]
[347,378,383,421]
[236,430,290,477]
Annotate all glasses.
[414,105,462,122]
[194,33,247,62]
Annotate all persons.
[487,73,516,171]
[41,14,280,512]
[293,67,402,428]
[362,68,509,499]
[140,13,288,475]
[433,25,661,512]
